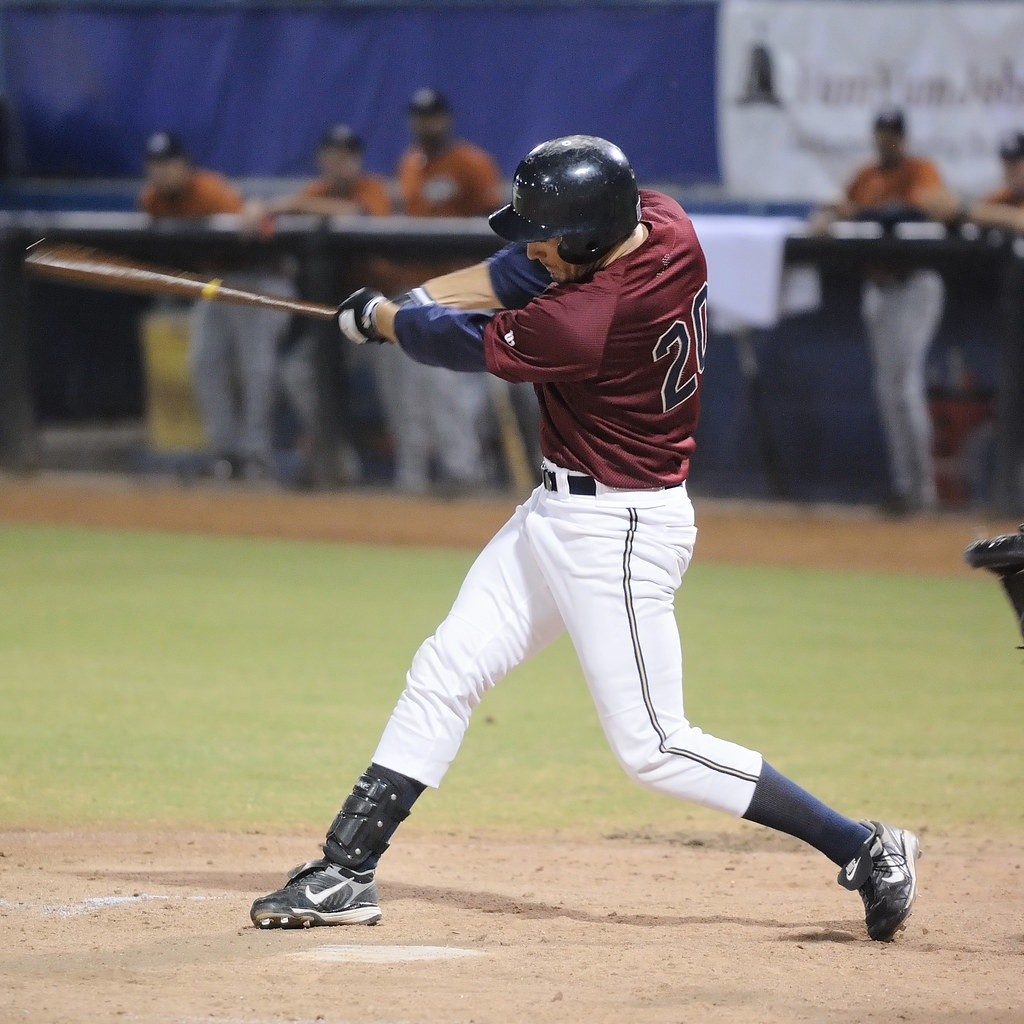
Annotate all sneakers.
[250,861,382,928]
[838,818,922,943]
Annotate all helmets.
[1000,132,1024,158]
[146,132,182,164]
[487,135,640,265]
[404,87,450,115]
[317,124,366,153]
[872,110,905,138]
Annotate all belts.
[542,468,681,496]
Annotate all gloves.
[334,288,388,347]
[392,288,433,316]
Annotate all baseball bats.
[23,244,339,324]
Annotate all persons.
[810,112,1024,506]
[249,136,918,941]
[142,91,505,489]
